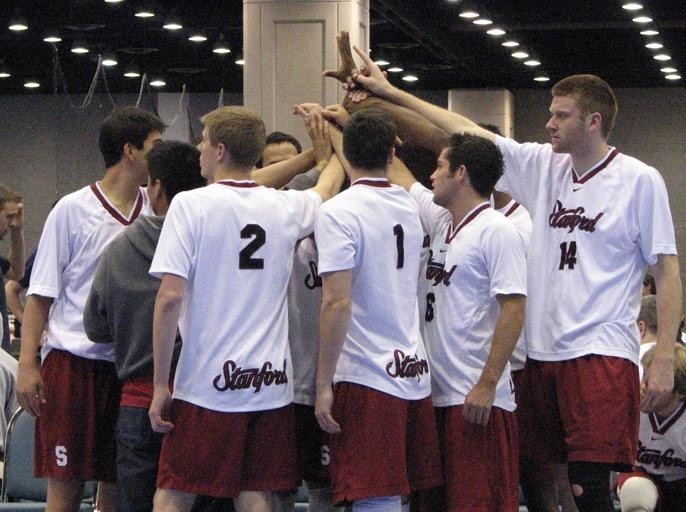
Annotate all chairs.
[0,404,97,511]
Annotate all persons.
[4,25,686,510]
